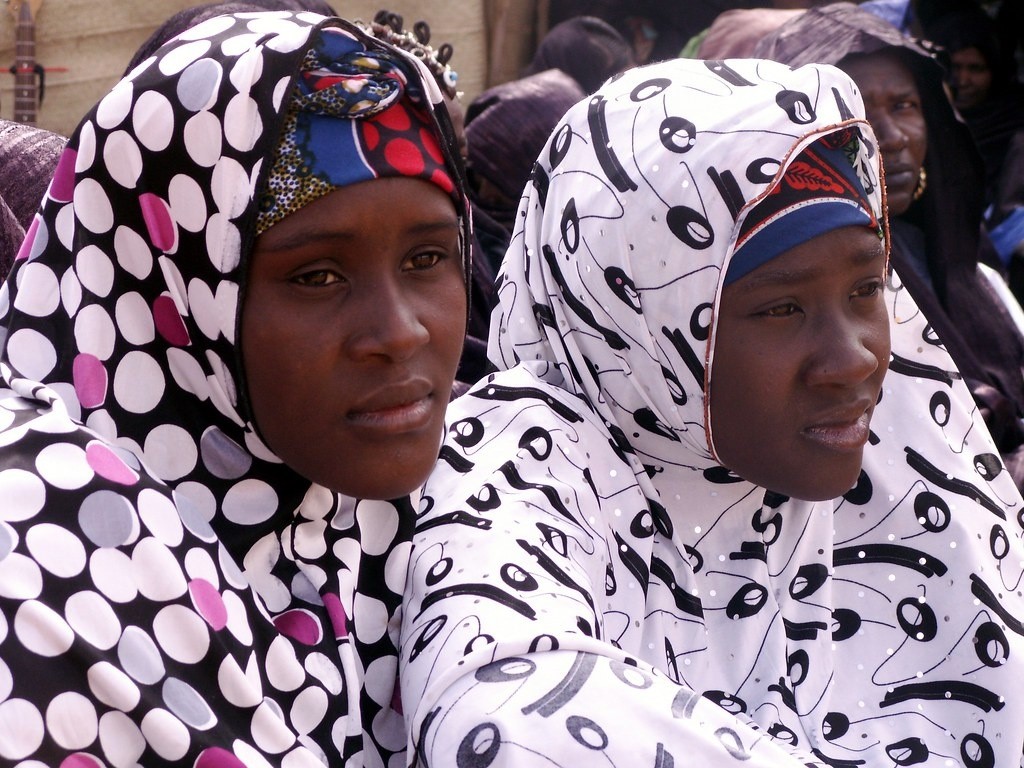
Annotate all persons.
[0,0,1024,768]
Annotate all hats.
[720,126,884,286]
[251,21,462,239]
